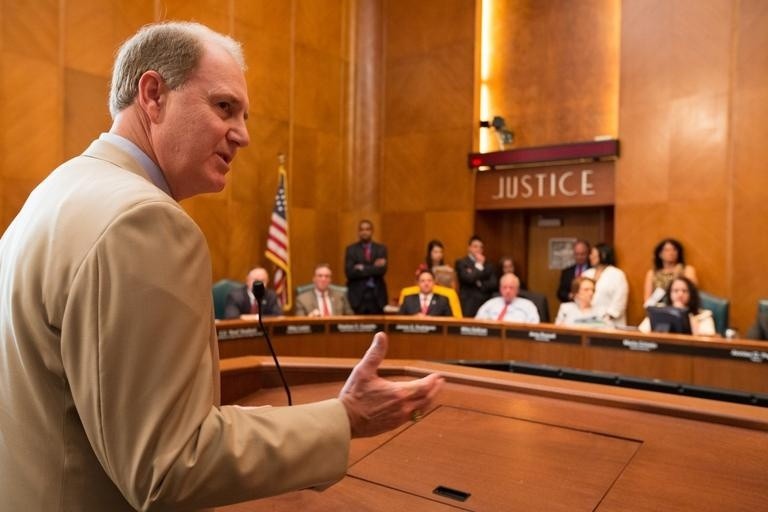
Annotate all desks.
[210,312,767,402]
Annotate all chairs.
[491,289,548,322]
[296,283,349,297]
[211,278,243,320]
[756,299,768,315]
[396,286,463,319]
[695,291,727,337]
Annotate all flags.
[262,154,293,317]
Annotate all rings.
[412,410,424,422]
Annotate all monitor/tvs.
[648,306,692,334]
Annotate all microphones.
[253,280,292,409]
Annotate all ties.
[320,291,330,316]
[421,295,429,314]
[497,302,510,322]
[362,245,372,282]
[249,295,258,315]
[576,264,583,278]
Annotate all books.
[432,263,452,288]
[643,287,665,308]
[608,320,638,331]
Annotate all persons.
[637,274,718,336]
[454,235,499,317]
[579,240,631,325]
[396,267,456,336]
[471,271,540,324]
[223,266,288,337]
[499,254,528,291]
[293,262,355,320]
[413,237,461,304]
[642,237,698,310]
[553,274,615,327]
[555,239,594,302]
[1,17,450,512]
[343,218,390,333]
